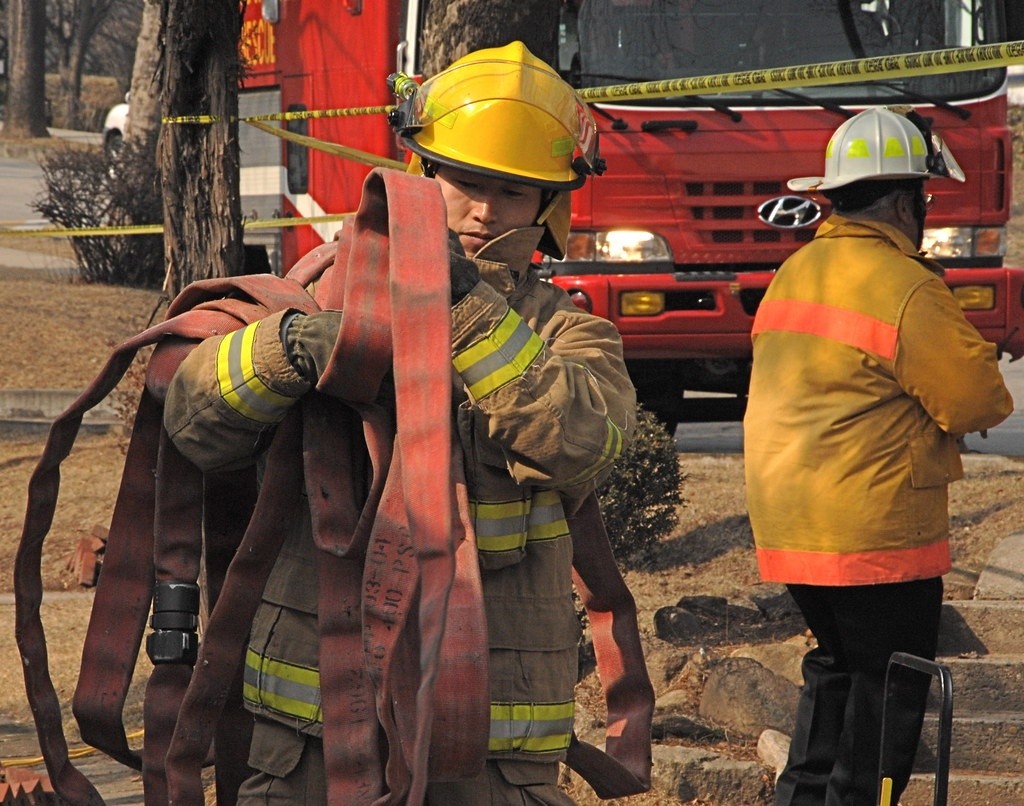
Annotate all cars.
[103,92,131,194]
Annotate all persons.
[742,104,1014,806]
[162,41,638,806]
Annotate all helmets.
[788,108,931,193]
[387,41,606,191]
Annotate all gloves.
[449,251,479,308]
[280,311,343,383]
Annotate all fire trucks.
[236,0,1024,437]
